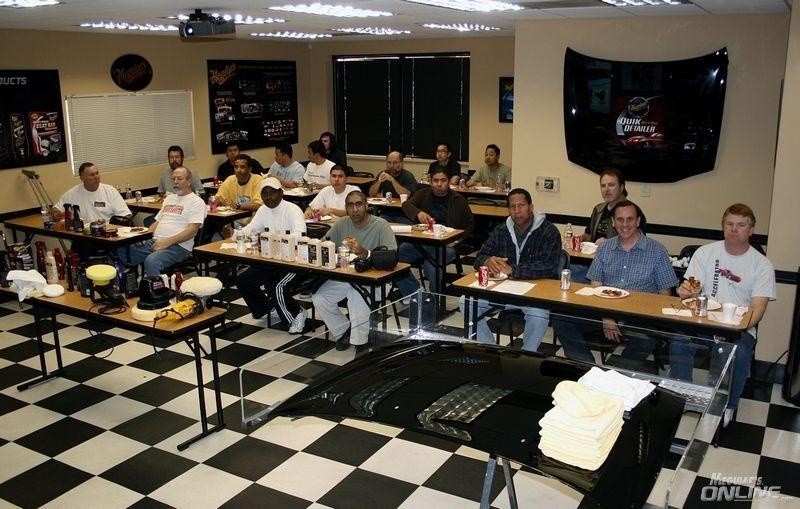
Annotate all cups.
[431,224,443,238]
[400,195,408,203]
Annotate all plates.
[476,271,508,282]
[581,240,596,247]
[593,286,630,299]
[680,297,723,310]
[366,196,387,206]
[320,214,333,221]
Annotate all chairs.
[0,172,759,509]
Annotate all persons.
[458,190,562,353]
[472,143,511,233]
[117,166,207,278]
[551,200,679,373]
[217,131,475,351]
[50,162,133,261]
[143,145,205,228]
[572,169,646,248]
[669,203,777,428]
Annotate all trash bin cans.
[740,358,780,403]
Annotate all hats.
[259,177,285,194]
[371,250,398,270]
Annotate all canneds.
[505,180,510,192]
[560,268,571,289]
[428,217,435,233]
[314,208,320,222]
[572,234,581,252]
[210,196,217,212]
[213,175,219,187]
[695,294,707,316]
[478,266,488,288]
[459,178,465,189]
[386,192,393,205]
[135,191,142,203]
[307,182,314,193]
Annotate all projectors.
[179,19,234,39]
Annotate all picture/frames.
[499,77,513,123]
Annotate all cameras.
[355,258,371,273]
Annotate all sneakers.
[354,343,370,360]
[289,306,308,334]
[336,326,350,351]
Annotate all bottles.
[232,225,350,271]
[563,223,573,251]
[297,175,304,195]
[420,168,429,184]
[31,242,182,308]
[113,182,132,200]
[495,174,504,191]
